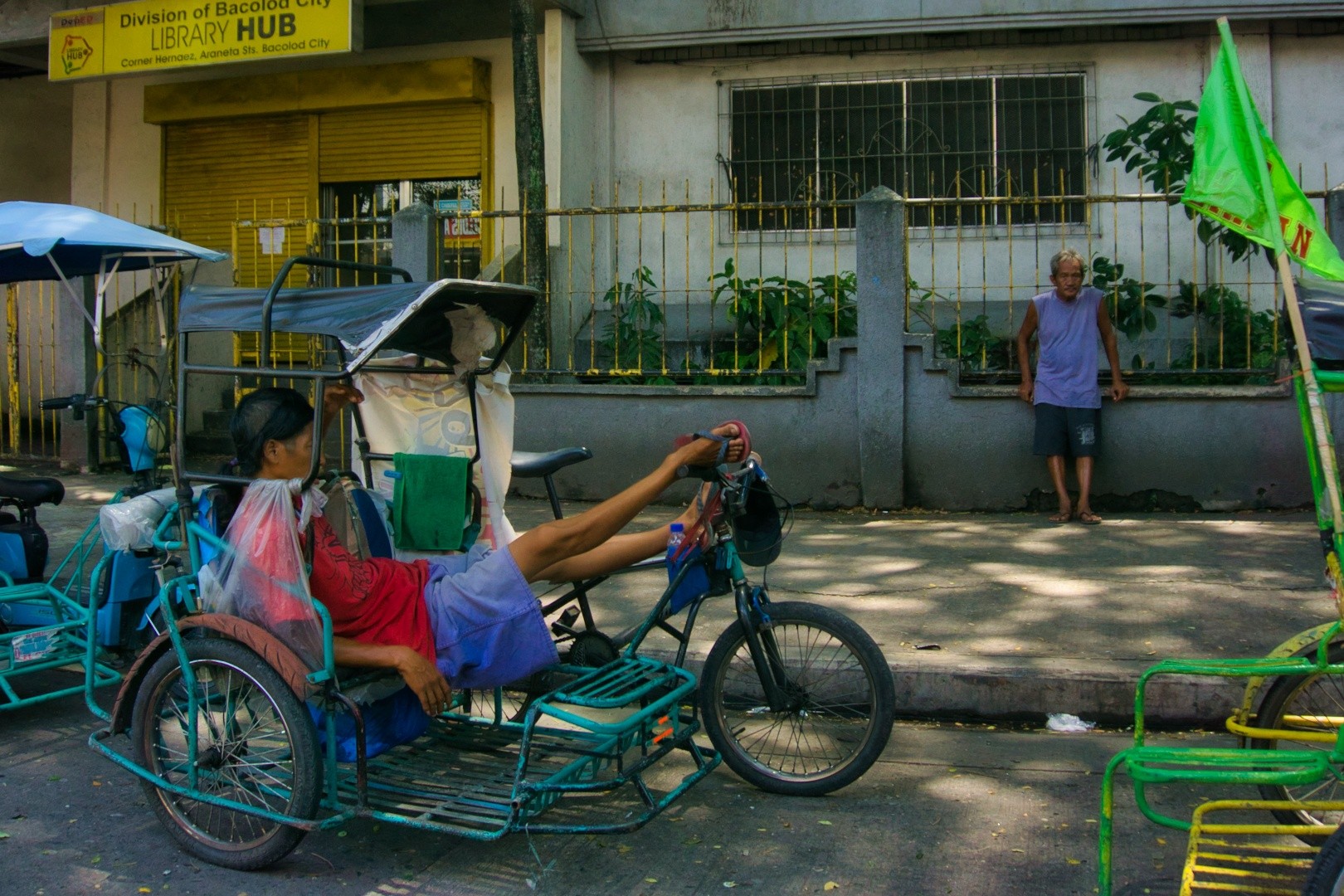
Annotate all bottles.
[668,523,690,562]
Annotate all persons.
[233,384,762,718]
[1017,248,1130,525]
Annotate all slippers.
[697,473,732,549]
[674,420,752,470]
[1077,512,1101,525]
[1048,509,1074,523]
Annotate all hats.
[723,457,782,567]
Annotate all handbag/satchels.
[663,471,733,616]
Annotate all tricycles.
[1099,288,1344,896]
[0,200,229,711]
[85,255,894,870]
